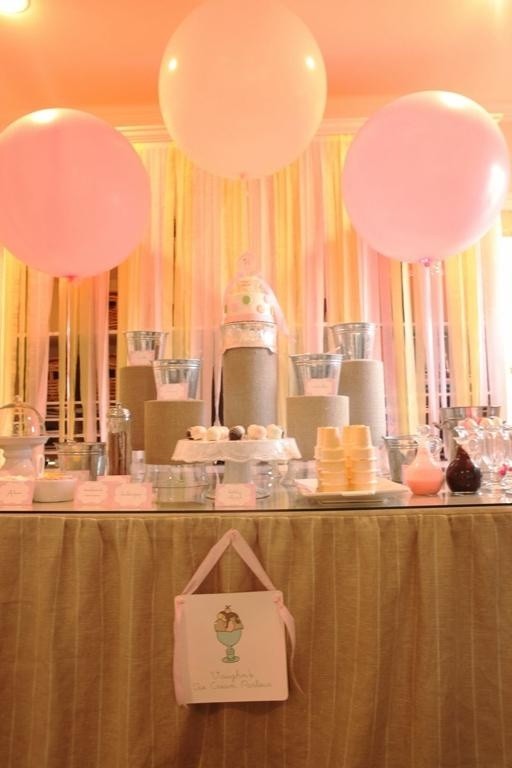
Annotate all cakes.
[221,273,283,325]
[463,415,502,432]
[187,425,285,442]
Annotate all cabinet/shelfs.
[7,256,129,475]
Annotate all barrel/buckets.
[381,435,419,483]
[123,330,170,361]
[53,441,106,480]
[329,321,376,359]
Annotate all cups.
[122,329,171,369]
[327,319,383,363]
[2,404,510,511]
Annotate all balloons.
[156,0,330,181]
[2,103,156,284]
[339,82,506,269]
